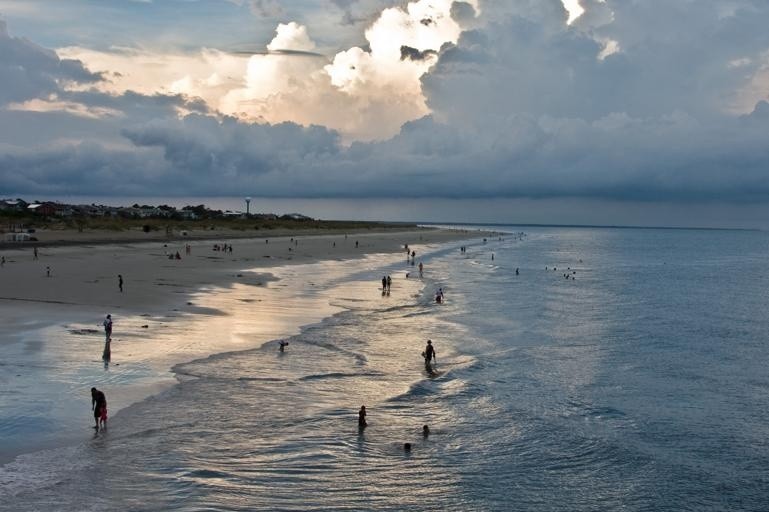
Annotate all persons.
[91,387,107,427]
[359,406,367,425]
[118,275,123,292]
[422,425,427,436]
[436,288,443,303]
[33,246,39,260]
[168,242,232,259]
[425,341,435,365]
[103,315,112,339]
[382,248,423,291]
[405,443,416,452]
